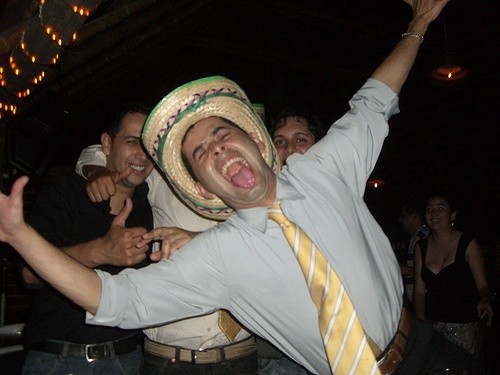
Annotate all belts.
[20,336,141,363]
[377,307,412,375]
[143,337,258,364]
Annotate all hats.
[142,76,281,220]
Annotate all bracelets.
[402,32,424,44]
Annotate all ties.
[266,203,382,375]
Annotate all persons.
[0,95,495,375]
[0,0,496,374]
